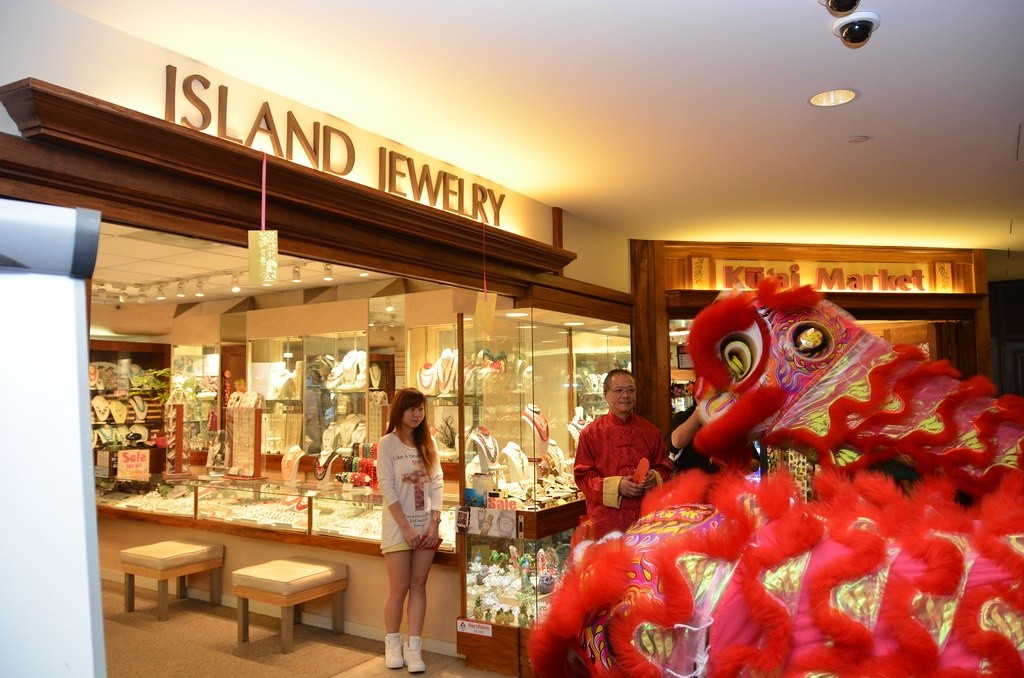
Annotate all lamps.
[231,276,241,292]
[385,297,394,312]
[292,266,301,283]
[156,286,165,300]
[324,263,333,281]
[138,287,147,304]
[176,282,185,298]
[195,281,205,297]
[118,286,127,303]
[97,283,107,299]
[263,273,271,287]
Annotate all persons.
[670,392,762,477]
[573,368,673,515]
[376,388,445,672]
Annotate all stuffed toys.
[526,276,1024,678]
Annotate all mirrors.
[219,312,246,458]
[368,297,405,443]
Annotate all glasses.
[607,387,637,395]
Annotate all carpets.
[100,578,384,678]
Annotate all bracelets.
[429,517,441,524]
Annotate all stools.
[119,539,224,622]
[233,556,347,654]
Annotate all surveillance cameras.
[832,11,880,44]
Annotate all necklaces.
[281,495,312,510]
[132,395,145,411]
[371,365,383,381]
[236,392,258,448]
[95,401,108,415]
[437,354,455,391]
[472,428,498,462]
[326,372,343,381]
[338,442,378,489]
[281,451,304,480]
[460,362,490,386]
[115,402,125,417]
[512,450,524,473]
[568,415,592,434]
[521,410,550,442]
[418,366,436,388]
[549,447,564,468]
[89,364,97,382]
[99,425,113,442]
[116,426,133,443]
[313,451,338,480]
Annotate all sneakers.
[385,632,403,669]
[402,636,426,673]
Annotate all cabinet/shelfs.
[89,339,171,491]
[170,290,521,486]
[369,352,395,404]
[456,487,586,678]
